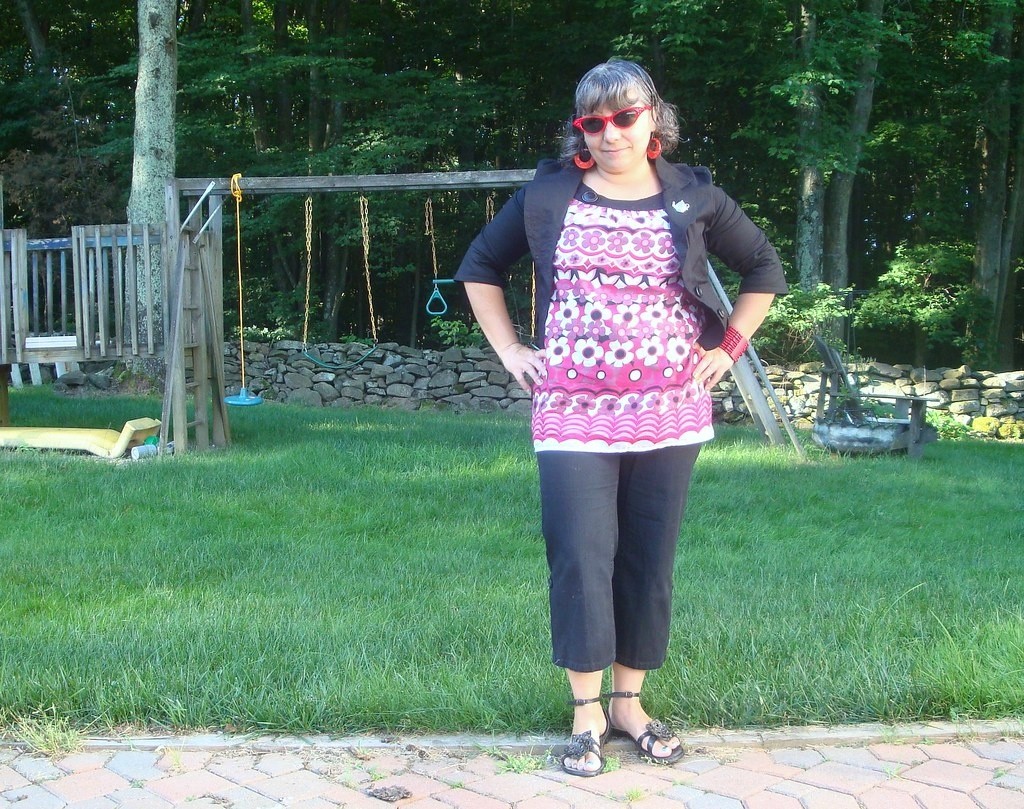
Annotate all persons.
[455,56,789,778]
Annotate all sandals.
[561,697,611,777]
[602,692,684,764]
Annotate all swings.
[302,196,379,369]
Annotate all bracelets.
[718,326,749,363]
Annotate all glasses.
[572,104,652,135]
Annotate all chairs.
[810,335,939,458]
